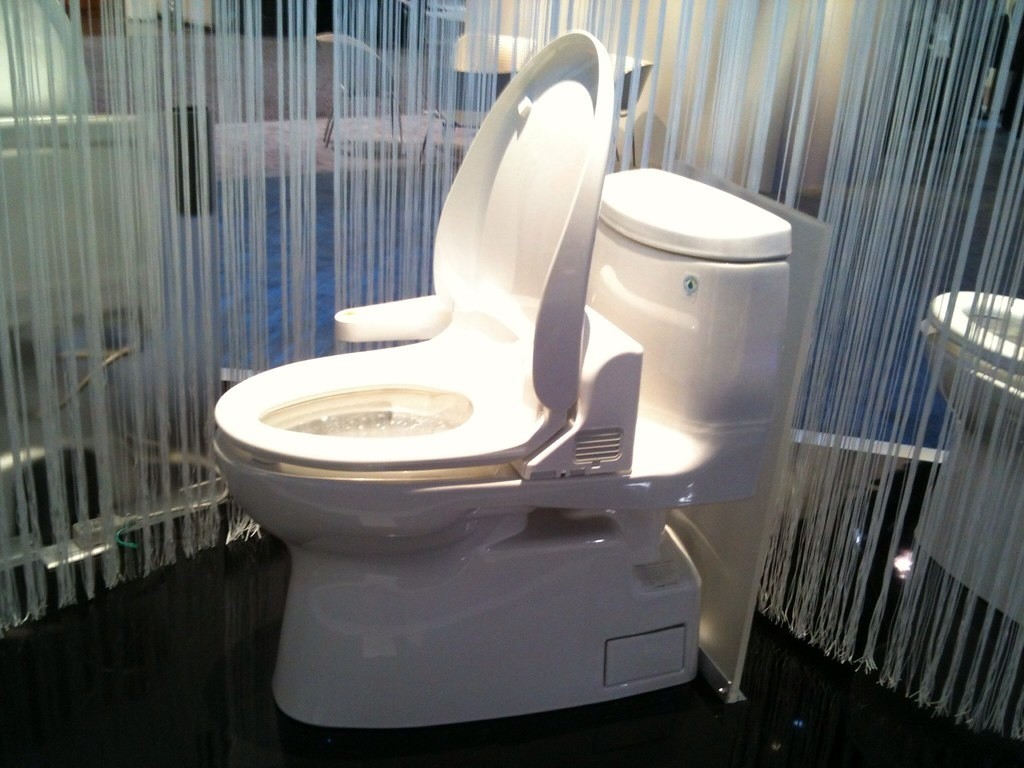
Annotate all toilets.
[915,291,1024,628]
[211,30,792,726]
